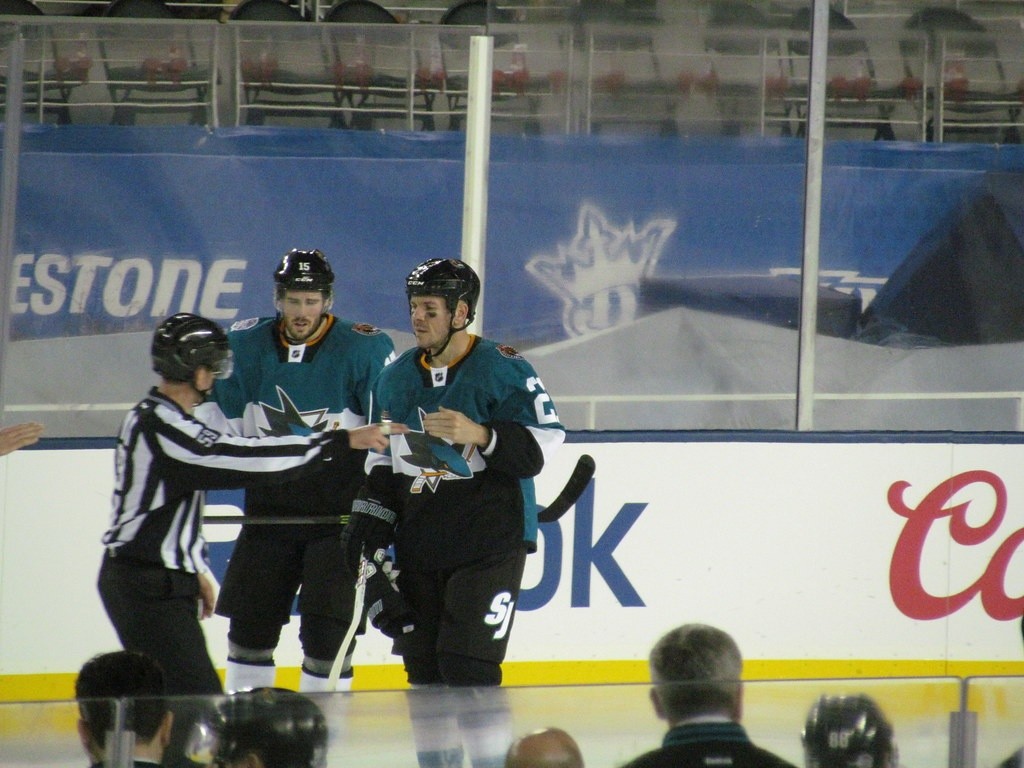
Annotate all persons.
[75,652,171,768]
[342,257,567,768]
[194,687,329,768]
[506,729,584,768]
[618,624,798,768]
[97,250,410,747]
[802,696,898,768]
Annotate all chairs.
[782,9,901,142]
[224,0,352,130]
[0,0,93,126]
[898,7,1023,145]
[698,0,794,140]
[95,0,223,126]
[439,1,541,140]
[557,1,680,139]
[323,0,446,132]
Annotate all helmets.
[273,248,334,317]
[801,696,893,768]
[405,257,481,316]
[151,312,234,383]
[183,686,329,768]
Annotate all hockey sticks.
[201,452,595,526]
